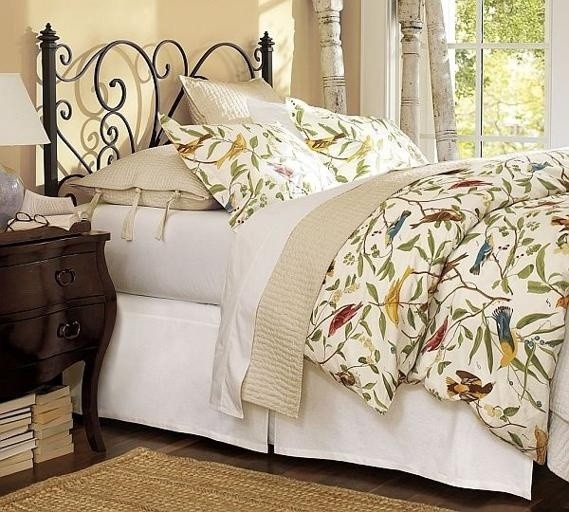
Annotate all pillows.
[245,100,302,140]
[160,122,337,228]
[285,96,427,184]
[63,141,222,209]
[180,78,285,124]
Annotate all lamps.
[0,73,52,234]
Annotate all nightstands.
[0,229,117,453]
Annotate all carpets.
[0,446,459,512]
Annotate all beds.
[37,24,569,499]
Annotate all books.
[0,384,74,477]
[7,189,87,231]
